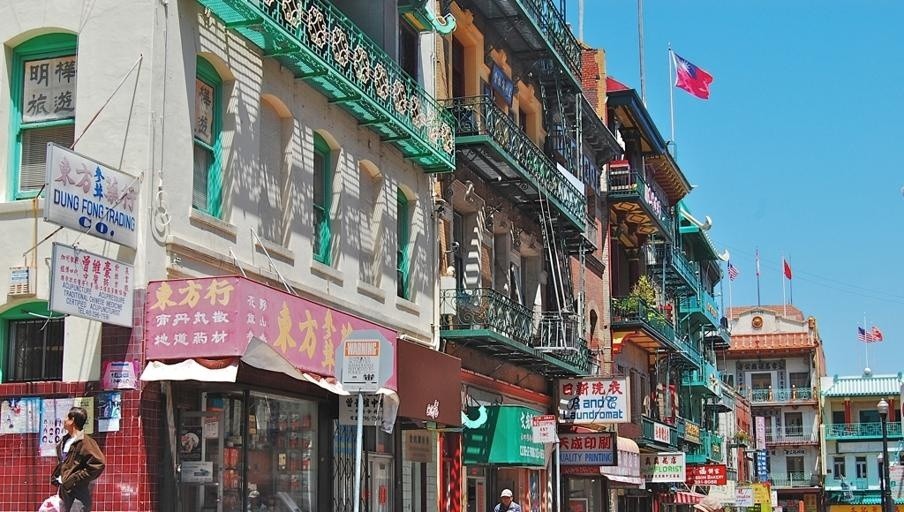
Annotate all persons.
[494,488,521,512]
[693,495,724,512]
[50,406,106,511]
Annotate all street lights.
[877,451,886,511]
[876,396,893,510]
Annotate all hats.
[500,488,513,498]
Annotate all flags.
[858,326,872,343]
[755,415,766,451]
[755,450,768,482]
[728,260,739,282]
[888,460,904,505]
[670,48,713,100]
[872,325,883,343]
[783,259,792,280]
